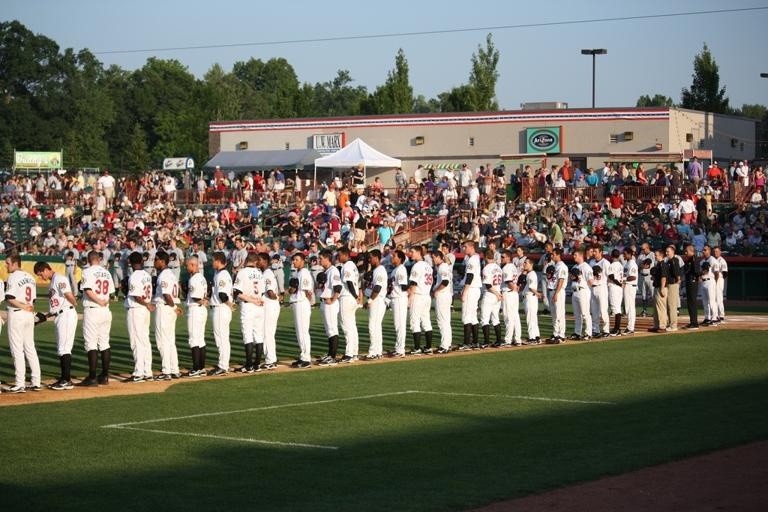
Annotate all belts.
[54,306,73,316]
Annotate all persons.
[1,157,768,394]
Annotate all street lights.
[580,47,607,108]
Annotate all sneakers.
[648,317,724,333]
[454,328,634,351]
[0,361,279,392]
[292,346,452,368]
